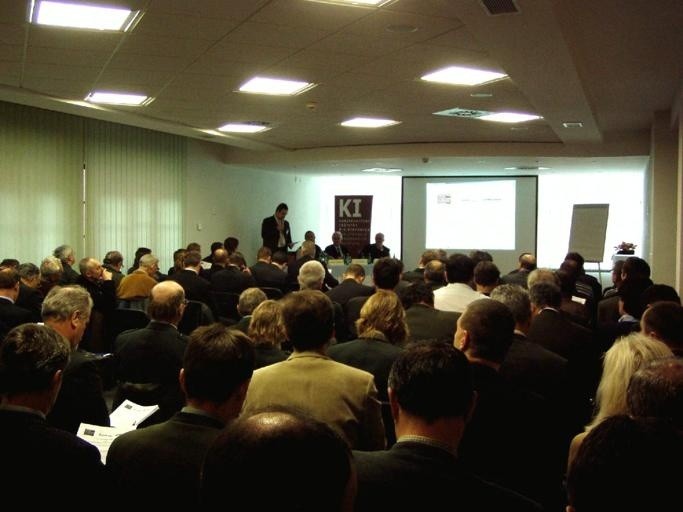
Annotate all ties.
[336,247,343,257]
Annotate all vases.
[617,250,635,254]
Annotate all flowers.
[613,242,637,252]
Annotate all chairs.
[107,287,284,335]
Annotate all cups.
[319,252,374,265]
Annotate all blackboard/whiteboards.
[568,204,609,263]
